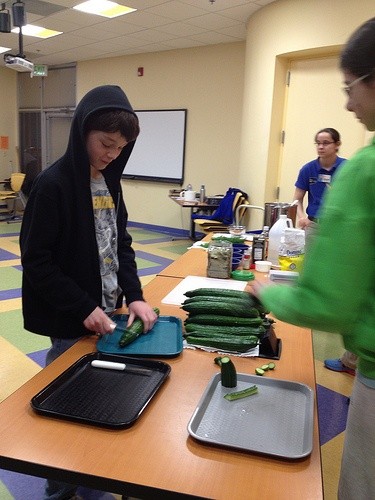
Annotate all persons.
[294,128,348,231]
[324,351,358,376]
[245,19,375,500]
[19,85,157,500]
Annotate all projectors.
[6,57,34,73]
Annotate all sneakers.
[324,359,355,376]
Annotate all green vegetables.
[198,236,247,248]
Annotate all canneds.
[206,240,233,278]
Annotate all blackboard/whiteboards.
[121,109,186,184]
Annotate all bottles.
[267,214,294,267]
[278,229,306,272]
[188,184,192,191]
[200,185,205,202]
[242,225,269,269]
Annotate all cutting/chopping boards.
[161,275,248,307]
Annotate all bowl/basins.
[227,225,246,235]
[231,243,249,271]
[254,260,272,273]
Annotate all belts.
[308,215,321,224]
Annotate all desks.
[0,232,325,500]
[168,195,220,242]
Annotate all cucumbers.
[220,357,275,401]
[118,307,160,348]
[180,288,276,352]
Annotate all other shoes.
[69,486,116,500]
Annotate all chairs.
[194,192,250,235]
[0,173,25,224]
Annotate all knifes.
[92,360,152,373]
[109,323,140,337]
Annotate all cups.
[180,191,196,201]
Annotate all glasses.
[314,141,337,146]
[342,71,375,95]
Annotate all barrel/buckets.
[264,202,298,229]
